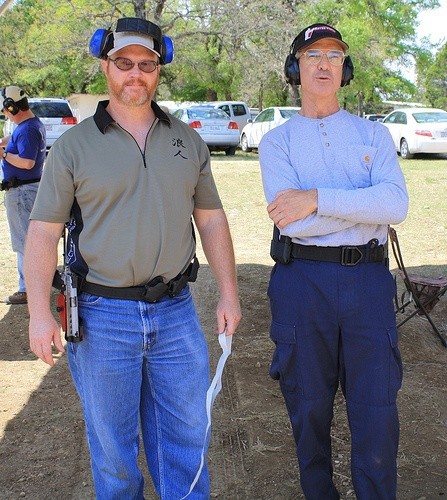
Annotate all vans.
[207,100,251,130]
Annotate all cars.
[364,108,447,160]
[240,107,301,153]
[172,105,241,157]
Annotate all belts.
[0,177,41,190]
[288,240,383,267]
[79,263,199,304]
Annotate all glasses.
[108,57,159,72]
[300,49,347,66]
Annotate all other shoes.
[5,291,28,304]
[52,270,66,291]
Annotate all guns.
[55,265,80,338]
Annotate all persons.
[0,85,47,304]
[257,23,411,500]
[21,19,241,500]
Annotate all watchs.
[2,151,9,160]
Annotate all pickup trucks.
[0,96,79,154]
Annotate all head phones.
[284,35,354,87]
[89,18,175,64]
[1,87,20,116]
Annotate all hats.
[291,23,349,59]
[100,30,164,57]
[0,86,26,111]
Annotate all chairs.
[392,114,447,124]
[387,224,447,349]
